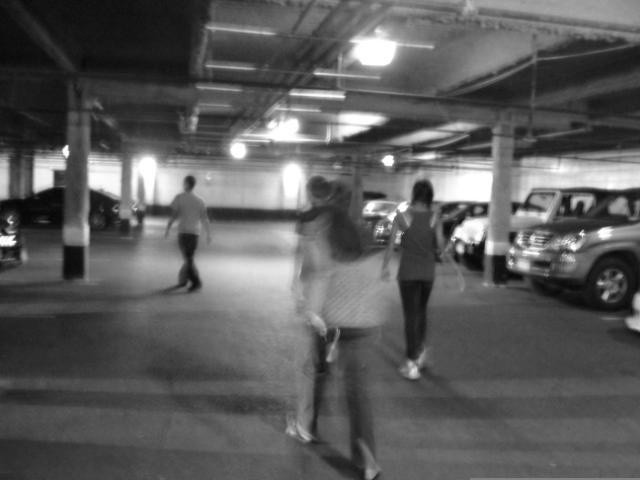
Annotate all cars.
[0,185,146,271]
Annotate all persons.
[281,177,395,479]
[376,178,469,381]
[164,172,214,291]
[295,176,331,318]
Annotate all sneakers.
[398,345,430,383]
[284,409,317,445]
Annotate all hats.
[294,174,353,223]
